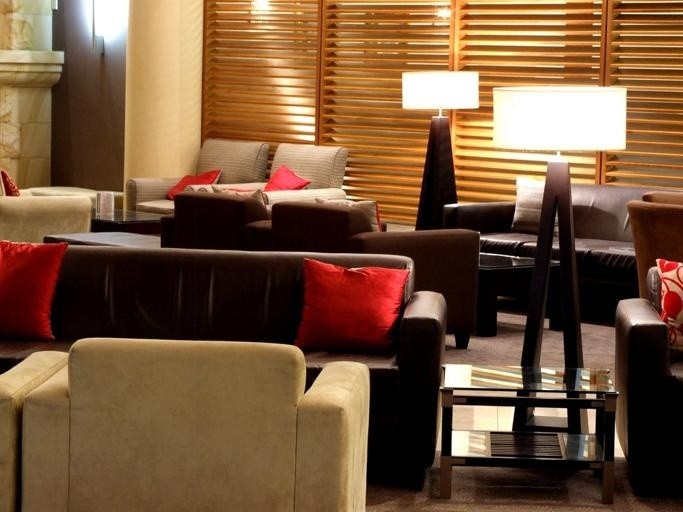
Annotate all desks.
[89,209,168,239]
[43,229,162,249]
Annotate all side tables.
[440,364,620,505]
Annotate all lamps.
[493,86,626,368]
[402,70,479,230]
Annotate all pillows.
[314,193,383,234]
[167,169,221,200]
[263,163,311,193]
[645,266,662,311]
[0,237,69,341]
[655,258,683,351]
[210,183,268,206]
[296,252,412,360]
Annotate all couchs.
[0,338,376,512]
[157,182,481,349]
[444,178,683,328]
[127,138,352,220]
[615,200,683,500]
[0,241,440,475]
[0,137,122,243]
